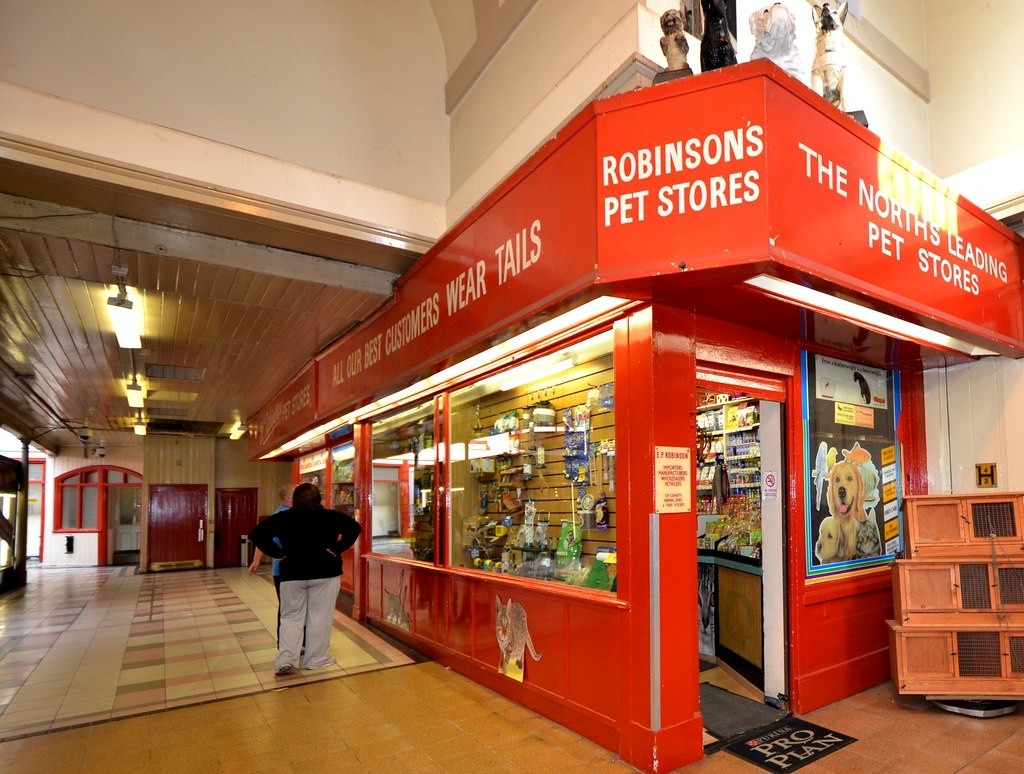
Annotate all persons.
[245,484,307,657]
[247,483,362,676]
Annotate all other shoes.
[306,657,336,669]
[274,664,295,674]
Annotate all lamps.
[133,409,147,436]
[229,424,247,440]
[106,284,142,349]
[126,374,144,408]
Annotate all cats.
[493,595,541,674]
[384,586,413,630]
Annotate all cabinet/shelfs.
[329,456,354,519]
[696,396,761,531]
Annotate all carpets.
[720,716,859,774]
[699,682,787,738]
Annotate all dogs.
[814,518,842,565]
[851,371,872,405]
[828,463,867,562]
